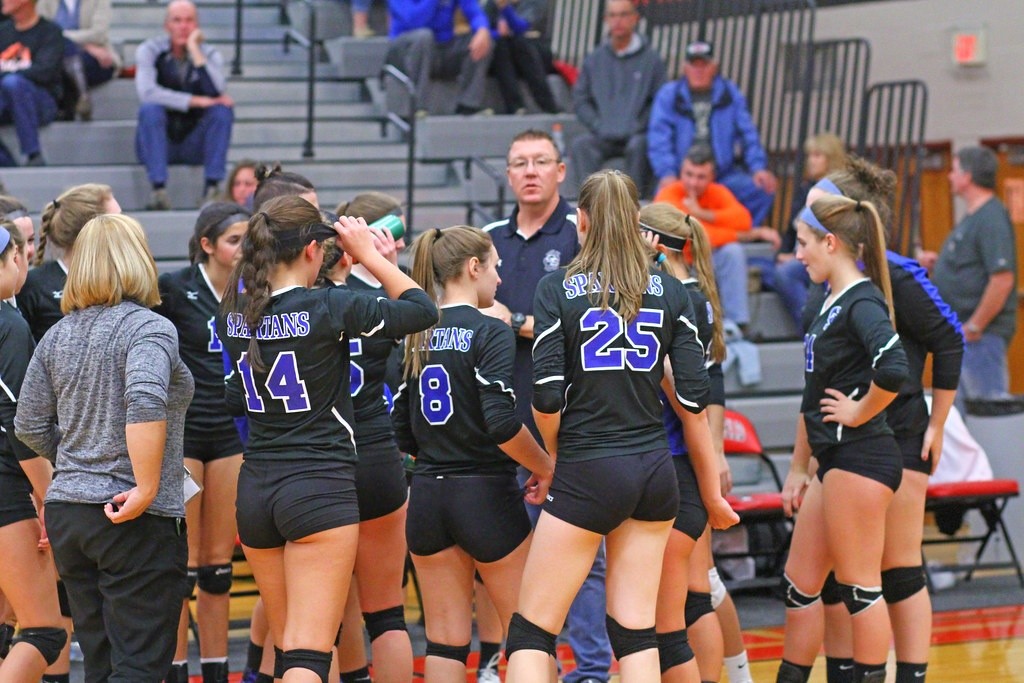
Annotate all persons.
[773,193,909,683]
[0,0,1024,683]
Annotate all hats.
[686,41,713,62]
[961,148,998,176]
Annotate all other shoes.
[78,94,92,118]
[25,154,45,166]
[475,651,503,683]
[197,186,219,207]
[148,188,171,211]
[722,318,744,342]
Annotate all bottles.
[350,215,404,265]
[552,124,565,157]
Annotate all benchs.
[0,0,807,453]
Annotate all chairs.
[714,410,796,558]
[922,479,1024,595]
